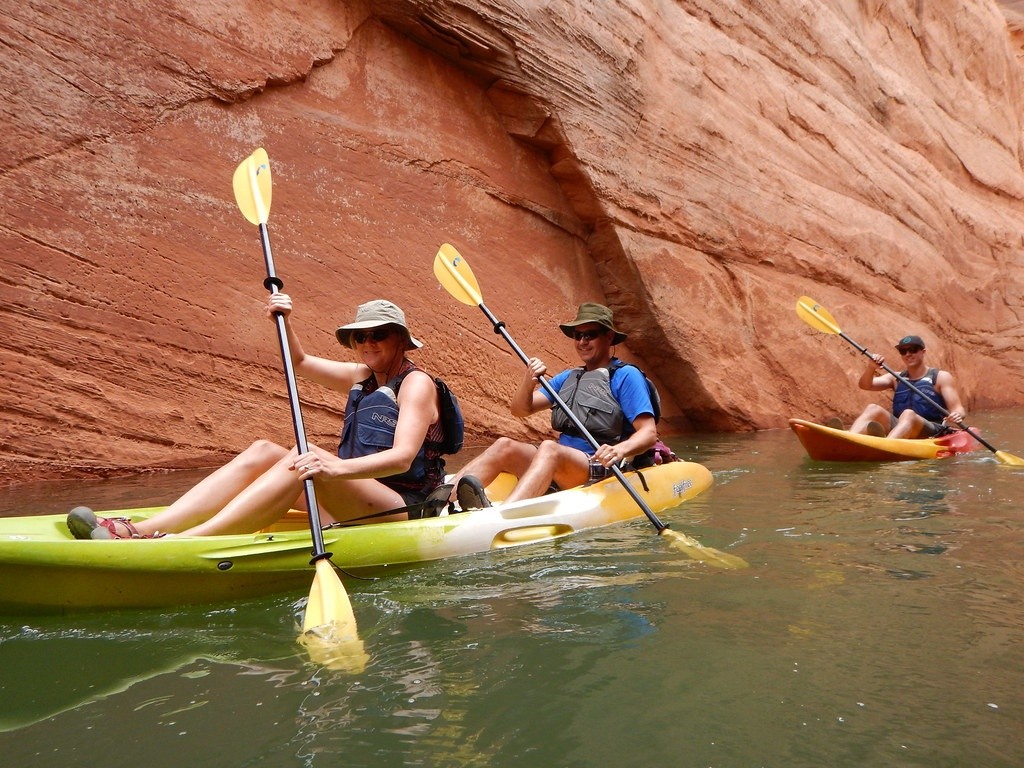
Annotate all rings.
[305,465,309,471]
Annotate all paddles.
[794,294,1024,467]
[231,145,360,648]
[431,241,674,537]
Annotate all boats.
[787,416,987,462]
[1,453,717,613]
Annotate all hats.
[895,336,925,350]
[335,298,423,351]
[559,302,627,347]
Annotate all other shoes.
[68,507,165,540]
[866,422,885,438]
[828,417,844,430]
[456,475,493,512]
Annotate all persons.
[67,292,444,540]
[448,302,657,510]
[828,335,965,439]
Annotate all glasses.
[899,347,922,355]
[572,327,611,340]
[352,327,403,344]
